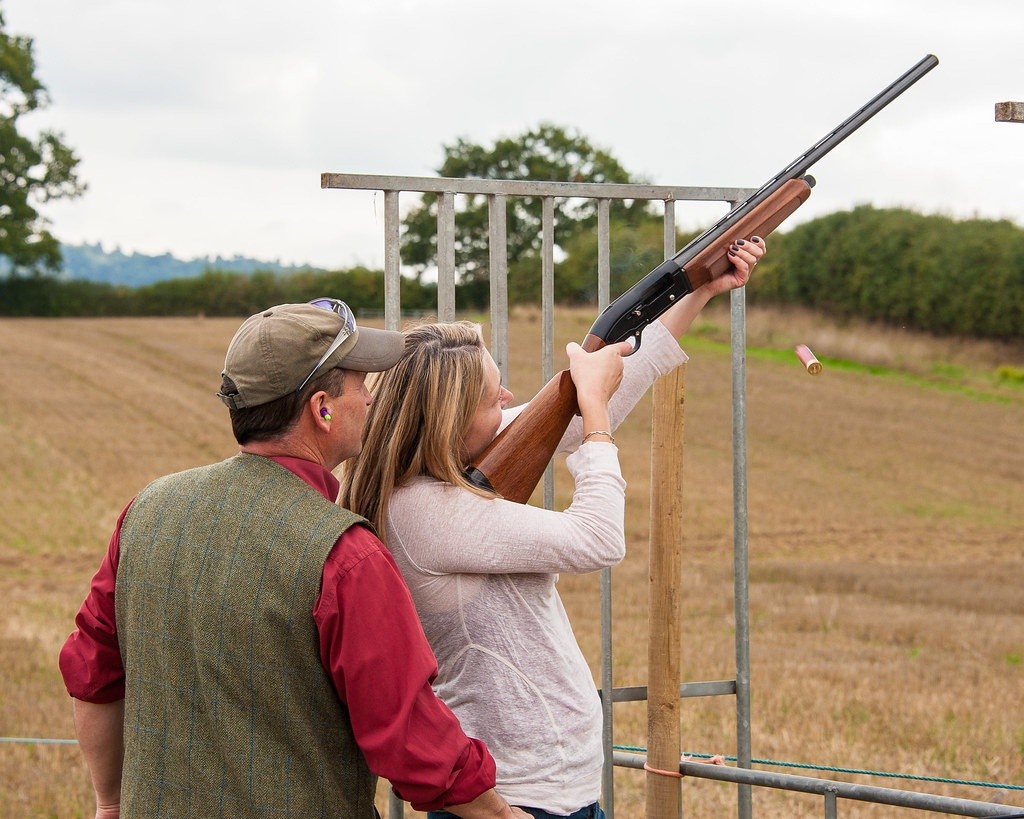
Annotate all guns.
[462,52,941,505]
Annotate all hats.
[218,303,402,410]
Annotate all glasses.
[299,298,358,390]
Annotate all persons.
[58,304,534,819]
[337,235,767,819]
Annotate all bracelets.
[581,430,615,447]
